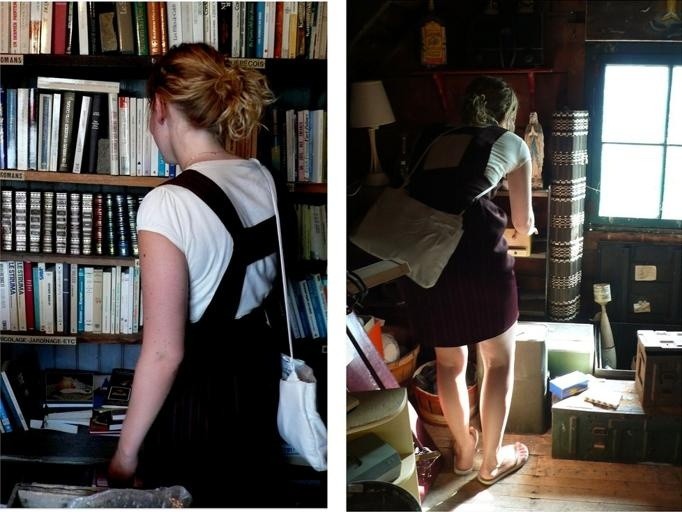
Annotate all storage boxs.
[551,330,682,465]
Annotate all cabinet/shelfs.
[490,183,552,322]
[0,51,326,352]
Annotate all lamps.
[350,81,396,185]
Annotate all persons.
[523,111,545,191]
[349,72,540,487]
[107,40,328,508]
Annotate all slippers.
[453,428,479,476]
[477,443,529,486]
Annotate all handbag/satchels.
[348,185,465,290]
[277,353,328,473]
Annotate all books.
[0,76,182,176]
[220,96,326,183]
[1,189,144,257]
[280,352,307,459]
[0,347,137,509]
[2,2,326,59]
[1,259,144,346]
[283,198,328,339]
